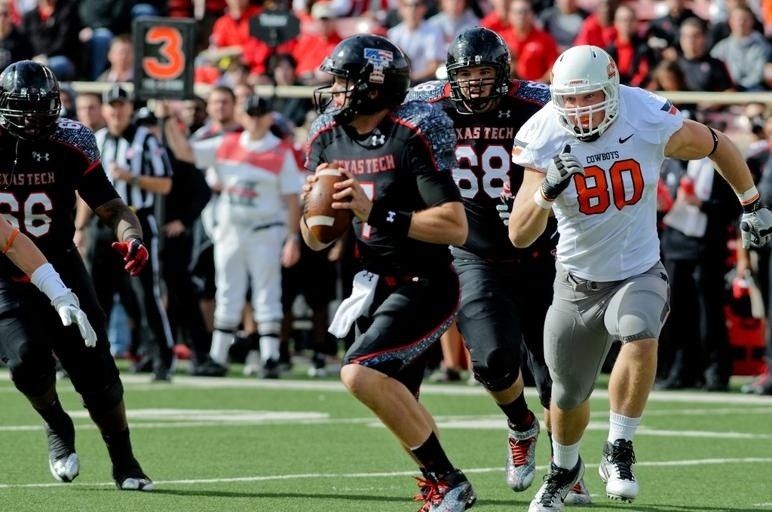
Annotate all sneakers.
[409,479,476,511]
[522,455,586,510]
[110,466,151,490]
[41,420,79,485]
[259,361,280,382]
[600,440,639,497]
[505,421,541,492]
[194,362,226,377]
[563,473,590,502]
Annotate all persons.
[260,54,316,136]
[0,0,36,72]
[79,0,163,77]
[574,0,617,49]
[72,87,175,380]
[95,36,136,82]
[279,240,340,375]
[497,0,561,84]
[644,0,711,44]
[0,61,153,489]
[510,45,772,512]
[163,95,306,378]
[648,17,740,92]
[188,85,259,376]
[302,35,476,512]
[655,110,741,391]
[22,0,85,80]
[0,214,98,348]
[478,0,508,31]
[386,0,447,86]
[292,1,346,85]
[75,94,132,359]
[652,61,686,90]
[539,0,592,45]
[57,87,73,118]
[603,5,653,87]
[125,107,192,363]
[426,0,480,41]
[711,7,772,92]
[400,27,591,507]
[430,323,477,384]
[711,0,765,44]
[248,0,301,88]
[211,0,271,67]
[149,95,210,359]
[733,117,772,394]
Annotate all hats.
[97,84,134,107]
[242,95,274,117]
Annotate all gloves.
[539,144,583,206]
[45,289,99,349]
[738,194,772,248]
[111,236,146,276]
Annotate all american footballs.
[303,163,354,243]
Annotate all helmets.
[313,36,412,125]
[0,58,64,142]
[549,43,622,138]
[445,27,510,119]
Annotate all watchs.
[132,173,140,187]
